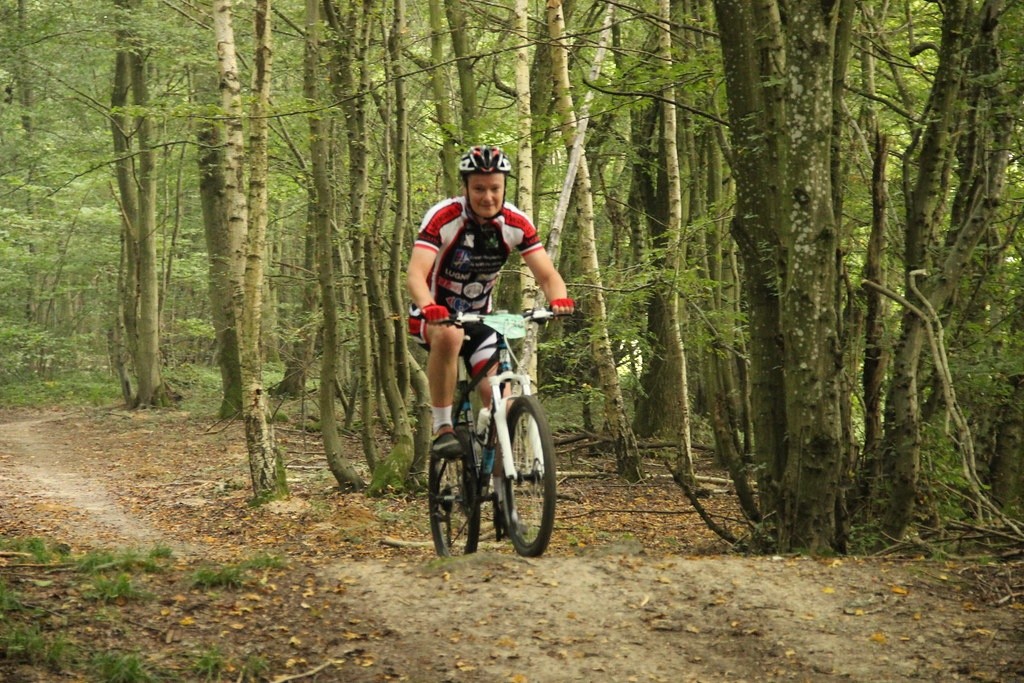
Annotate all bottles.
[477,407,491,445]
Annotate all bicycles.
[427,305,572,557]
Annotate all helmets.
[458,145,511,173]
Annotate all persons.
[407,145,575,535]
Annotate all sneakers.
[430,424,462,458]
